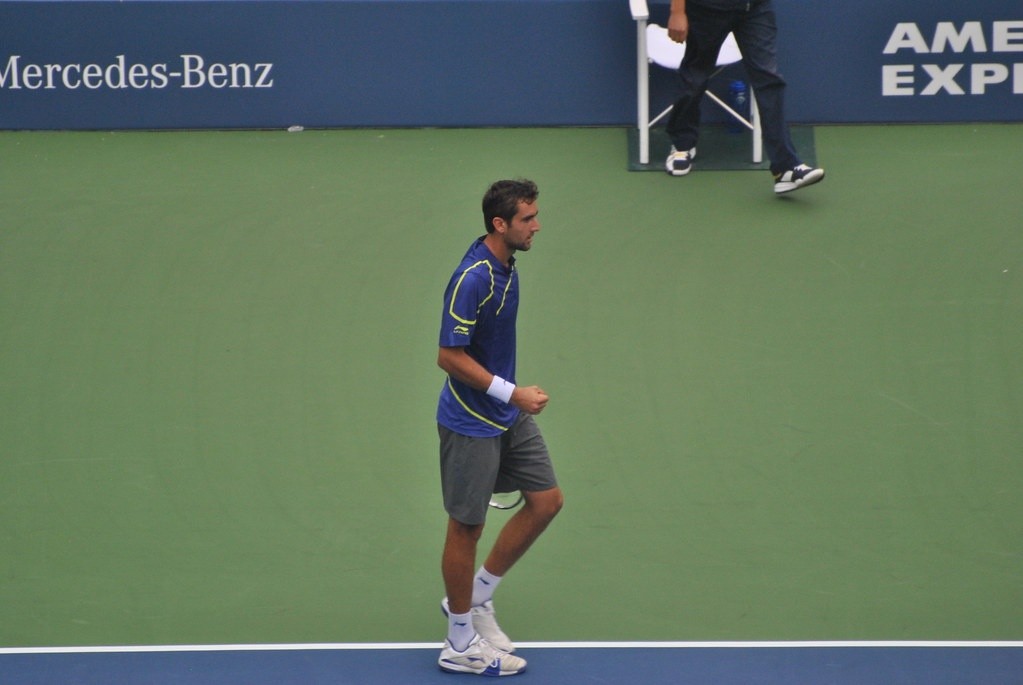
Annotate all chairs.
[628,0,764,164]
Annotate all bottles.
[727,80,749,134]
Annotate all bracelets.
[486,374,515,403]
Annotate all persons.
[436,176,563,676]
[666,0,825,194]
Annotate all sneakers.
[665,144,697,176]
[774,164,825,193]
[437,633,528,676]
[440,594,515,653]
